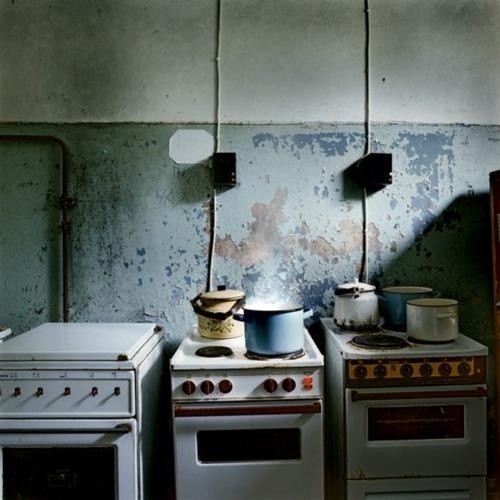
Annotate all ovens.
[345,384,488,500]
[174,403,324,500]
[0,418,139,500]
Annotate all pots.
[406,299,460,342]
[190,285,246,339]
[382,286,441,326]
[232,299,314,356]
[332,281,381,330]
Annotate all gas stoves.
[171,321,324,403]
[320,316,488,385]
